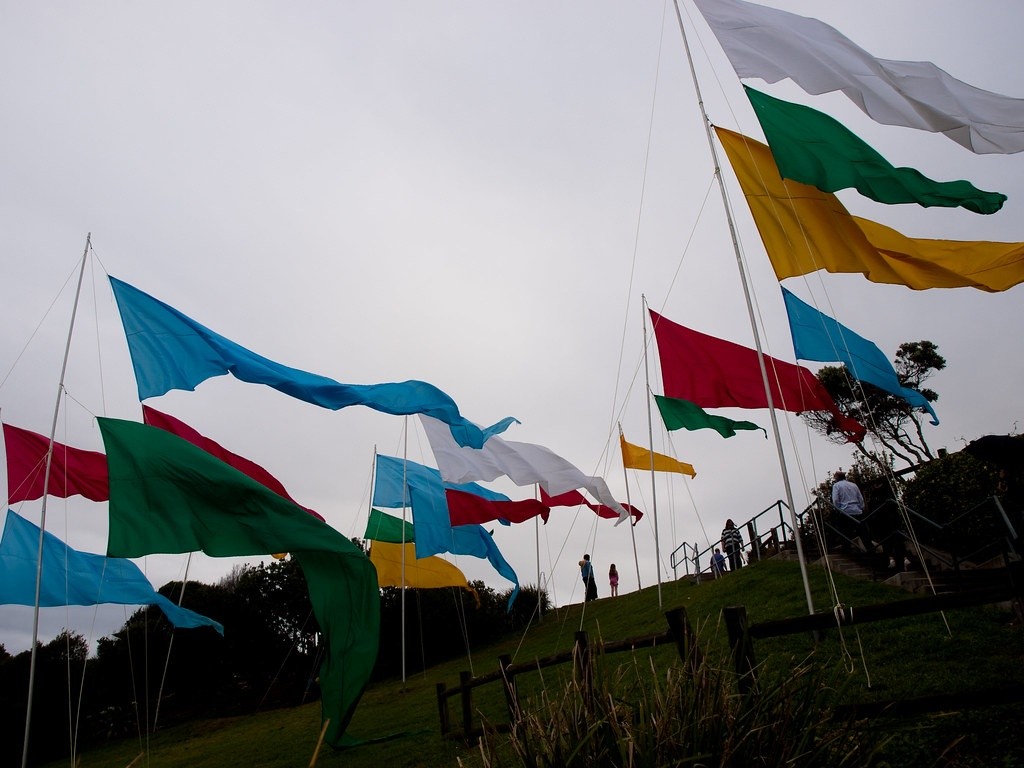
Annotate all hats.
[579,559,586,568]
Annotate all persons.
[609,564,618,597]
[721,519,745,572]
[867,483,908,582]
[581,554,598,602]
[746,536,766,565]
[832,471,865,564]
[710,548,727,580]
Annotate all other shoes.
[904,559,911,572]
[888,561,895,568]
